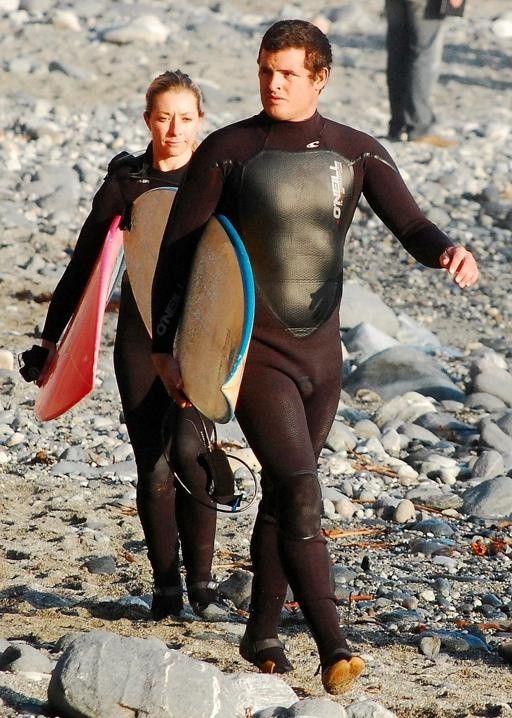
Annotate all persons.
[386,1,463,147]
[37,70,233,621]
[151,21,478,695]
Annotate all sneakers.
[191,588,229,620]
[239,636,292,673]
[153,579,185,621]
[325,655,364,697]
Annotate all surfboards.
[36,216,123,421]
[122,187,255,424]
